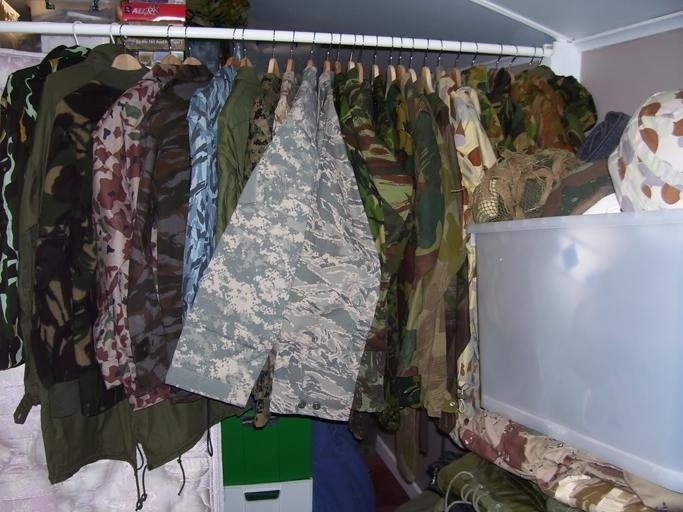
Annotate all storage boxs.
[222,410,315,511]
